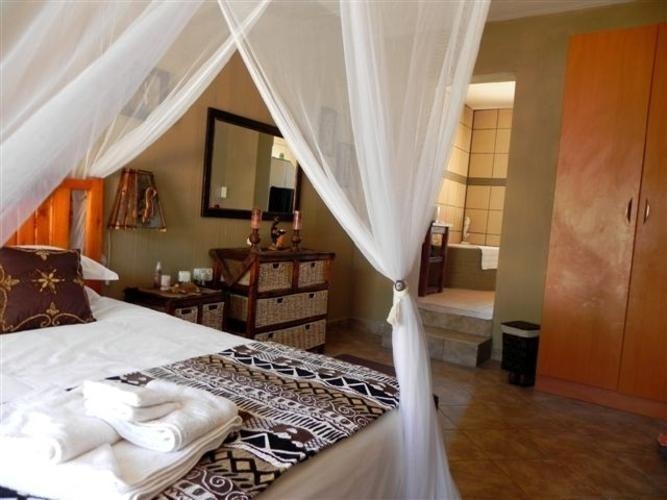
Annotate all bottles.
[176,267,212,290]
[154,262,171,292]
[433,205,441,223]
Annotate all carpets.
[335,354,395,376]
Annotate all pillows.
[0,245,119,334]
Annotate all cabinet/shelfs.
[208,248,336,350]
[125,286,223,330]
[419,222,450,297]
[533,18,667,424]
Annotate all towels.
[0,378,243,500]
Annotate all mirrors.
[200,106,302,221]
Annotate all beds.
[0,178,437,500]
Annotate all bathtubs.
[446,243,500,292]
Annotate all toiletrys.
[154,260,192,293]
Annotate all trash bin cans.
[500,321,540,387]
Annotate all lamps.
[107,169,166,288]
